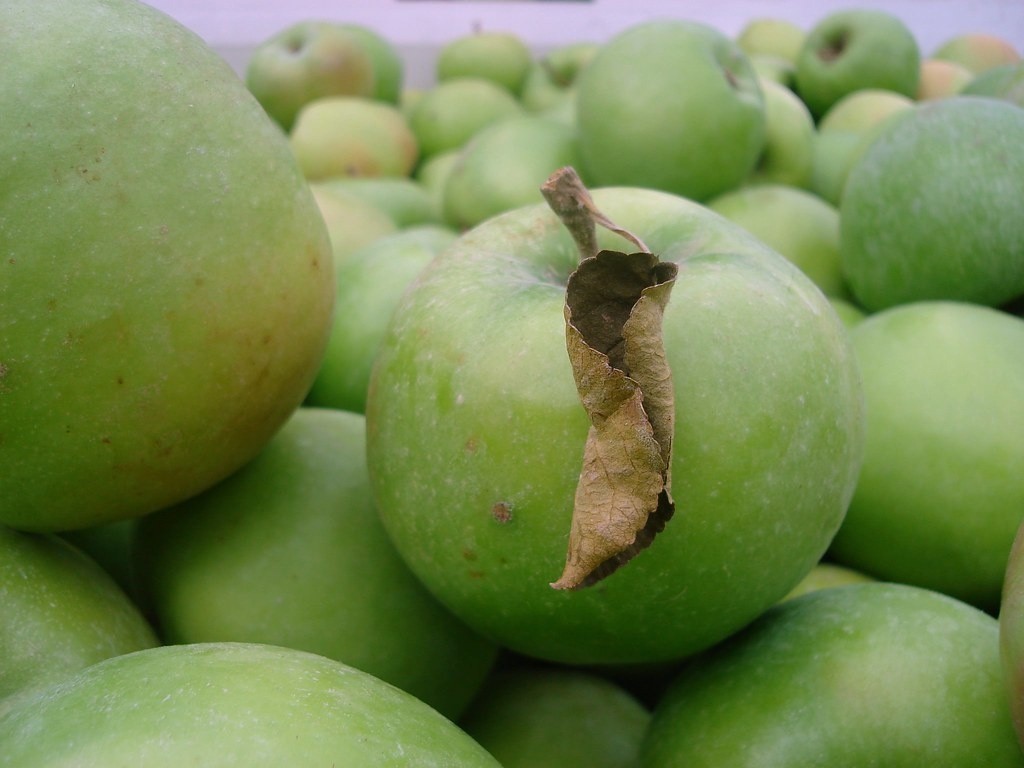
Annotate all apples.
[0,0,1024,768]
